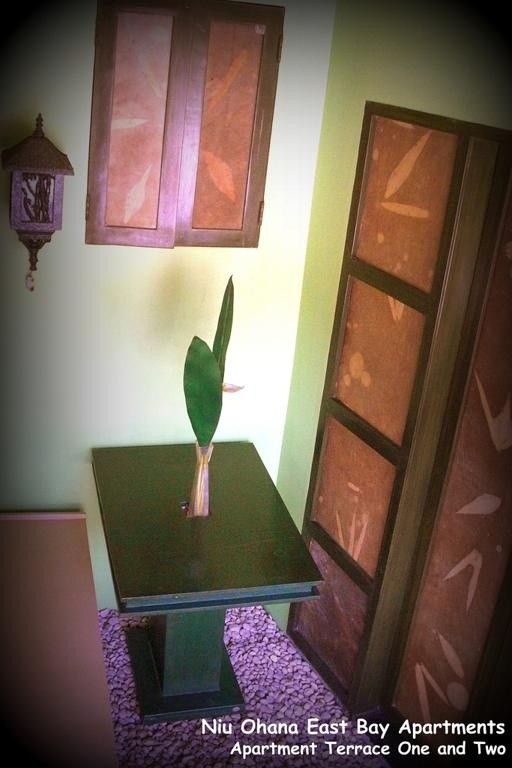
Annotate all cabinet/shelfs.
[283,96,512,768]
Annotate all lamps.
[0,112,75,290]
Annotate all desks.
[88,442,324,725]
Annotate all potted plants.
[181,273,246,517]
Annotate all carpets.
[95,598,388,768]
